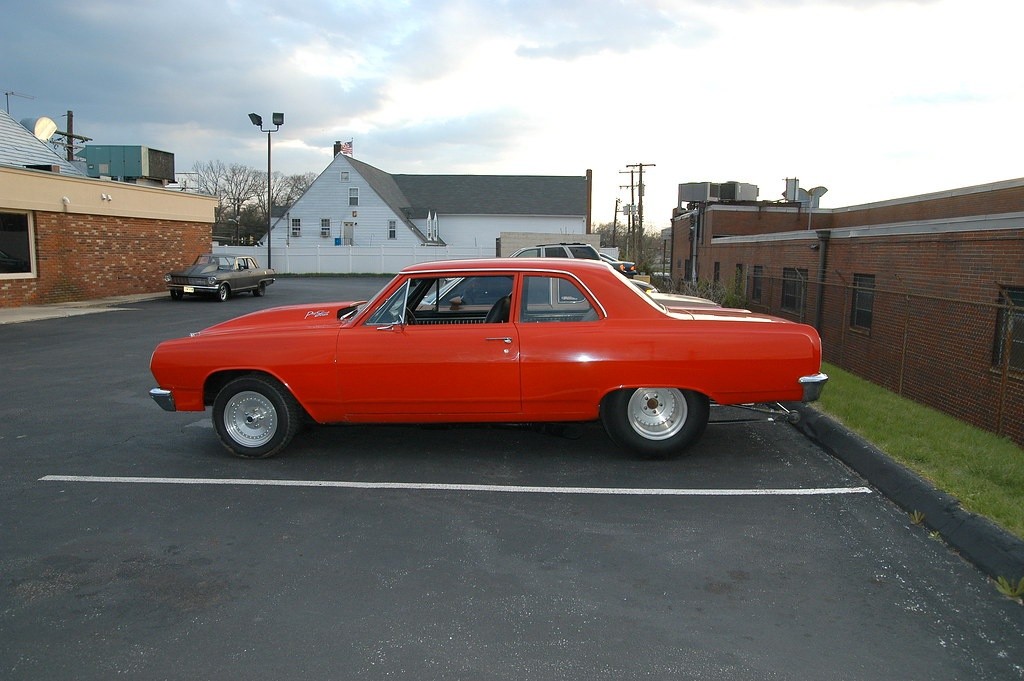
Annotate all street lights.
[247,112,284,271]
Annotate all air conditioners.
[291,231,299,237]
[320,230,330,237]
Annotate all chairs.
[484,295,511,324]
[232,263,240,270]
[508,291,512,301]
[581,308,599,321]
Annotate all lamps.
[810,244,819,249]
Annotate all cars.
[396,240,724,320]
[164,253,276,303]
[147,256,828,459]
